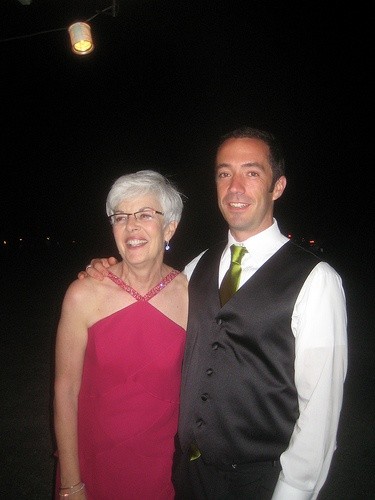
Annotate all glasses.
[108,210,164,225]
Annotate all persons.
[53,170,189,500]
[78,128,348,500]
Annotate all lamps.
[68,21,94,56]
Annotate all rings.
[86,264,94,274]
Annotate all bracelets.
[59,480,85,497]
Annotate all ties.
[190,244,246,461]
